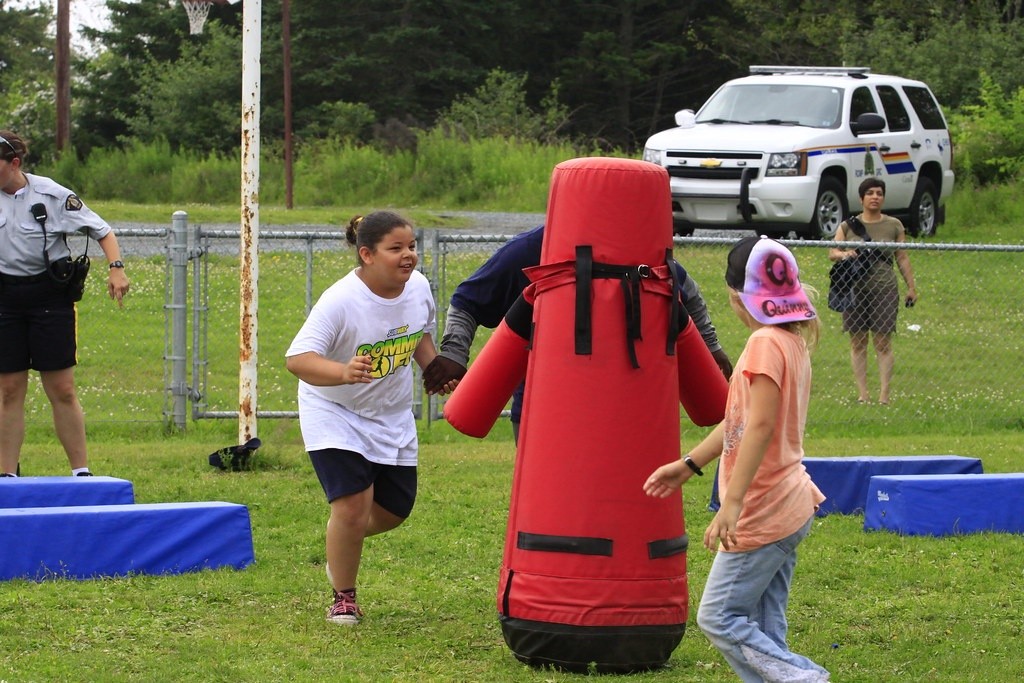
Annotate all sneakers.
[325,588,363,627]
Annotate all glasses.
[0,136,17,156]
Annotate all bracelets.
[683,455,703,477]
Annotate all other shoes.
[858,395,871,406]
[0,462,20,477]
[877,397,890,409]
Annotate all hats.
[725,235,817,325]
[209,438,261,471]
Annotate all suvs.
[641,65,955,240]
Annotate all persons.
[0,130,130,477]
[284,212,460,627]
[421,224,733,446]
[829,178,917,407]
[643,234,831,683]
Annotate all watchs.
[110,261,125,269]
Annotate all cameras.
[904,297,914,308]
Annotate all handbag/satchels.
[829,249,869,294]
